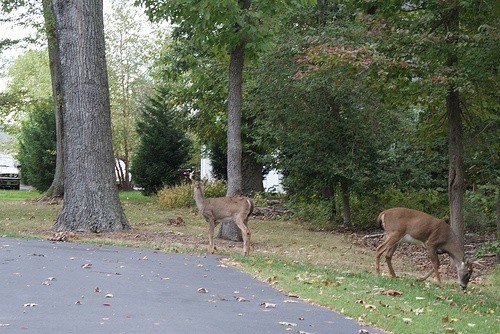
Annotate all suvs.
[0,154,22,190]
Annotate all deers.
[376,207,473,290]
[194,179,254,257]
